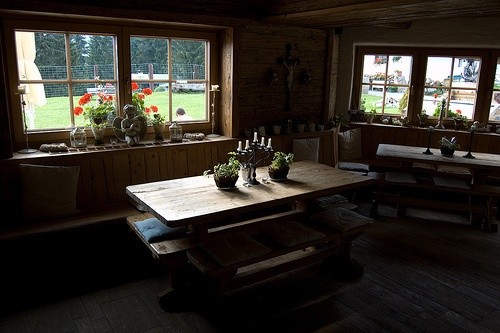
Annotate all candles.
[268,138,271,146]
[238,141,242,150]
[253,132,257,141]
[18,85,25,93]
[246,140,249,148]
[212,85,219,90]
[261,137,265,145]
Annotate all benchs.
[367,157,500,196]
[128,202,375,279]
[0,205,140,236]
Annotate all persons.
[121,103,144,146]
[174,107,194,121]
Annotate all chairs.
[332,126,370,173]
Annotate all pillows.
[337,127,363,161]
[293,138,319,162]
[18,163,82,221]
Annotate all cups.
[258,125,266,136]
[297,124,305,133]
[272,125,281,135]
[318,124,325,131]
[309,123,316,132]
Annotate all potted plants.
[214,151,295,188]
[90,122,107,144]
[152,113,165,140]
[366,109,377,123]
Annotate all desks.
[376,143,500,226]
[125,159,375,325]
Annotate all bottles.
[169,121,183,141]
[70,126,87,147]
[287,118,301,133]
